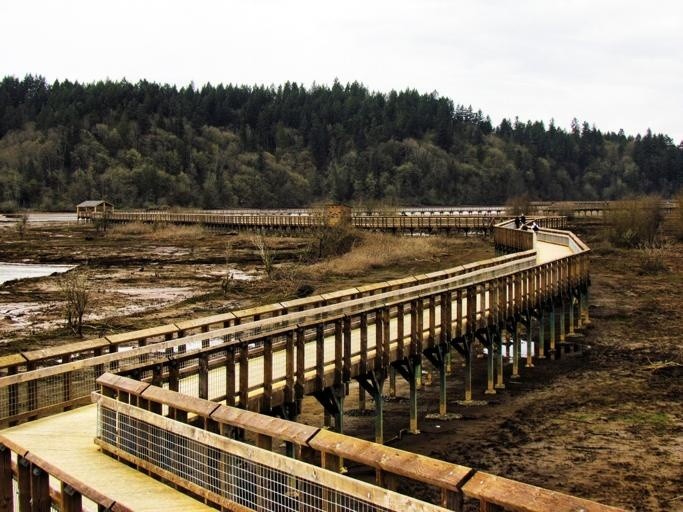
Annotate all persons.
[515,216,521,228]
[520,213,526,226]
[531,222,538,233]
[520,223,528,231]
